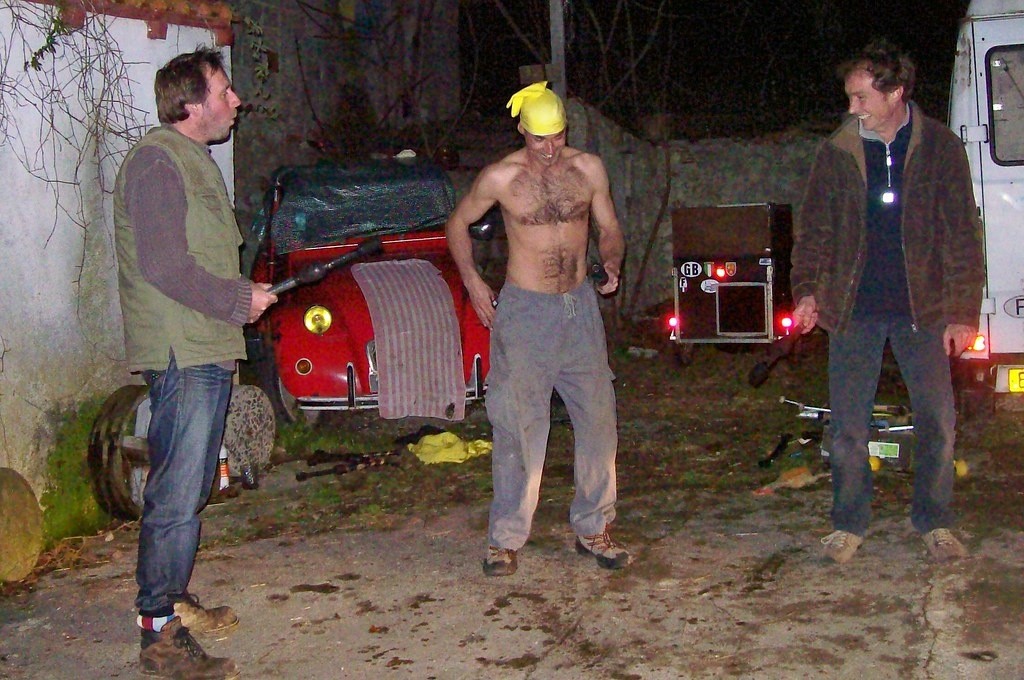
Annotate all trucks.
[935,0,1024,396]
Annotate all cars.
[242,162,501,426]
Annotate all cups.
[239,462,260,490]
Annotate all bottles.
[218,445,230,490]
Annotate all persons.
[445,81,631,578]
[790,36,985,563]
[114,47,278,680]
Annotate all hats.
[506,79,567,136]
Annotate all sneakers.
[173,588,239,632]
[921,527,971,562]
[821,530,863,563]
[575,523,632,569]
[138,616,241,680]
[483,546,517,576]
